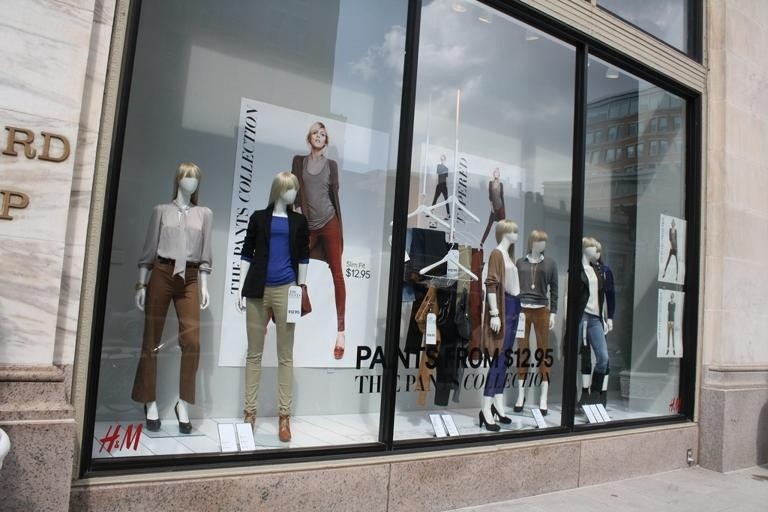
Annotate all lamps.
[526,29,539,40]
[450,1,467,12]
[480,11,493,23]
[605,67,619,79]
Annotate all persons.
[478,219,521,430]
[234,171,310,442]
[574,236,609,413]
[665,292,676,355]
[478,168,506,249]
[516,230,558,416]
[662,218,678,281]
[578,240,615,410]
[131,161,214,434]
[426,155,451,221]
[266,122,346,359]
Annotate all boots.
[577,388,588,407]
[598,390,607,407]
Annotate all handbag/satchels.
[272,284,312,323]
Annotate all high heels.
[515,395,526,413]
[174,401,193,434]
[478,410,500,432]
[143,402,162,432]
[539,397,548,416]
[278,415,292,443]
[244,410,256,431]
[335,332,346,360]
[491,403,512,424]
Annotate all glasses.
[441,158,445,161]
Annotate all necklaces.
[530,256,542,290]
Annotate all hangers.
[390,193,456,232]
[423,183,480,224]
[419,240,479,282]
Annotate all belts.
[158,256,199,268]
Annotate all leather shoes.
[425,212,433,217]
[673,350,676,355]
[666,349,669,355]
[662,272,666,278]
[444,215,450,220]
[676,275,678,280]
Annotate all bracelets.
[490,313,501,317]
[136,282,148,290]
